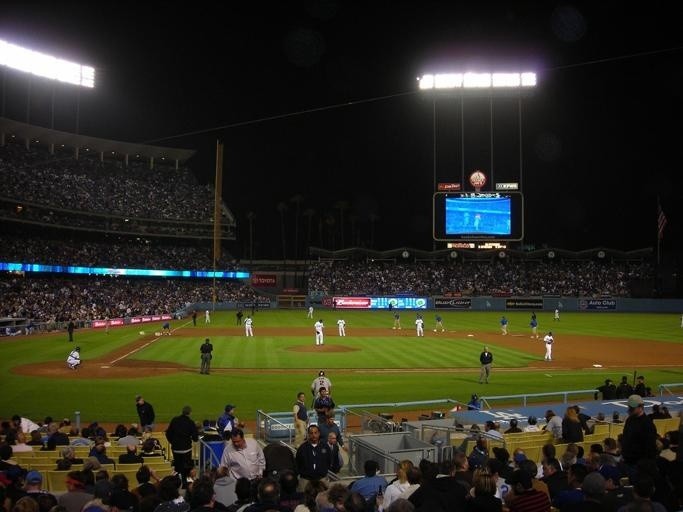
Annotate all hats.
[624,395,644,405]
[492,447,509,459]
[514,454,526,464]
[505,470,530,484]
[27,471,43,484]
[225,405,235,412]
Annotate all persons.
[66,347,81,370]
[388,302,445,338]
[500,309,562,340]
[304,252,682,297]
[1,137,273,342]
[479,346,492,385]
[544,332,555,361]
[200,339,214,375]
[306,299,346,347]
[1,372,682,511]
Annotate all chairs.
[451,417,681,465]
[0,430,202,493]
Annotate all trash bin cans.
[202,434,225,471]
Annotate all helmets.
[319,371,324,376]
